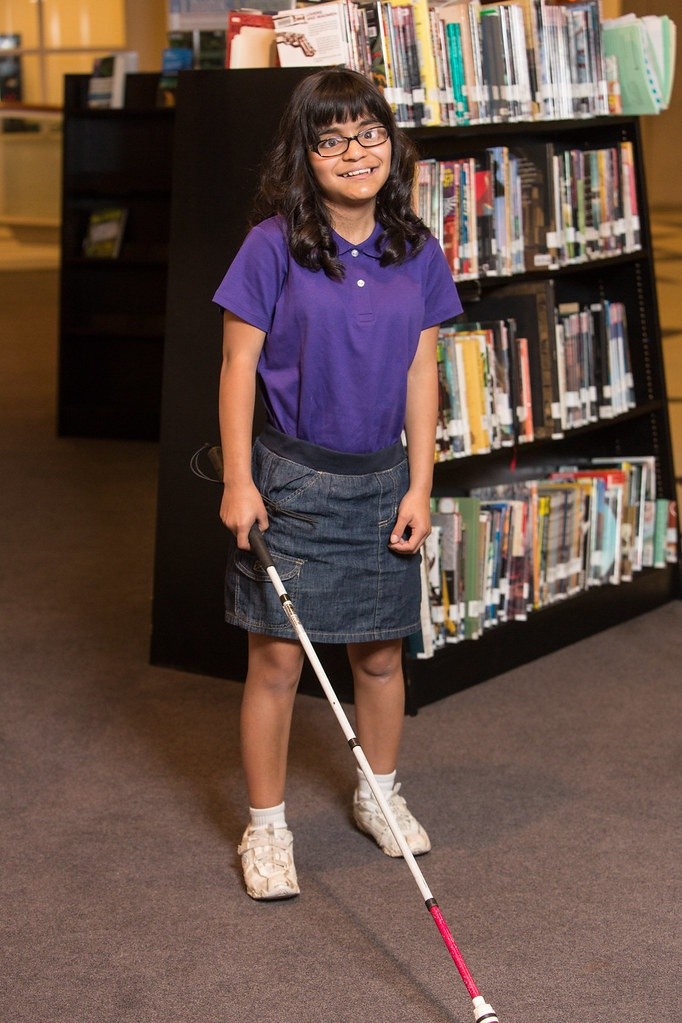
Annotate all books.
[84,208,128,259]
[224,0,677,128]
[158,48,194,109]
[89,55,126,110]
[415,450,678,659]
[433,298,637,461]
[408,141,642,283]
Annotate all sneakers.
[238,822,300,899]
[353,787,433,858]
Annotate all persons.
[212,68,466,901]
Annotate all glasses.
[310,125,390,157]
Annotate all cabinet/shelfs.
[146,66,682,719]
[53,74,162,447]
